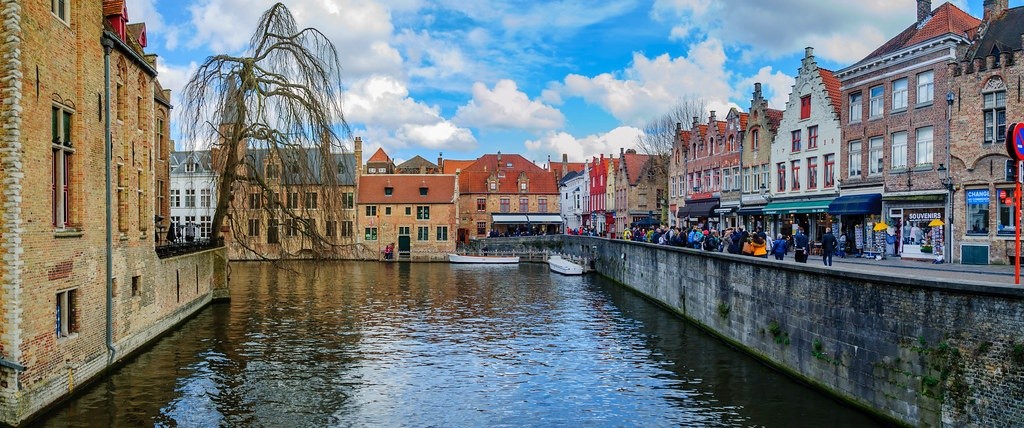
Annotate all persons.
[564,221,618,239]
[903,220,913,243]
[908,222,921,244]
[837,229,848,259]
[485,226,548,237]
[623,225,812,264]
[382,241,395,260]
[820,227,838,266]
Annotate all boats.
[447,253,521,264]
[546,255,583,275]
